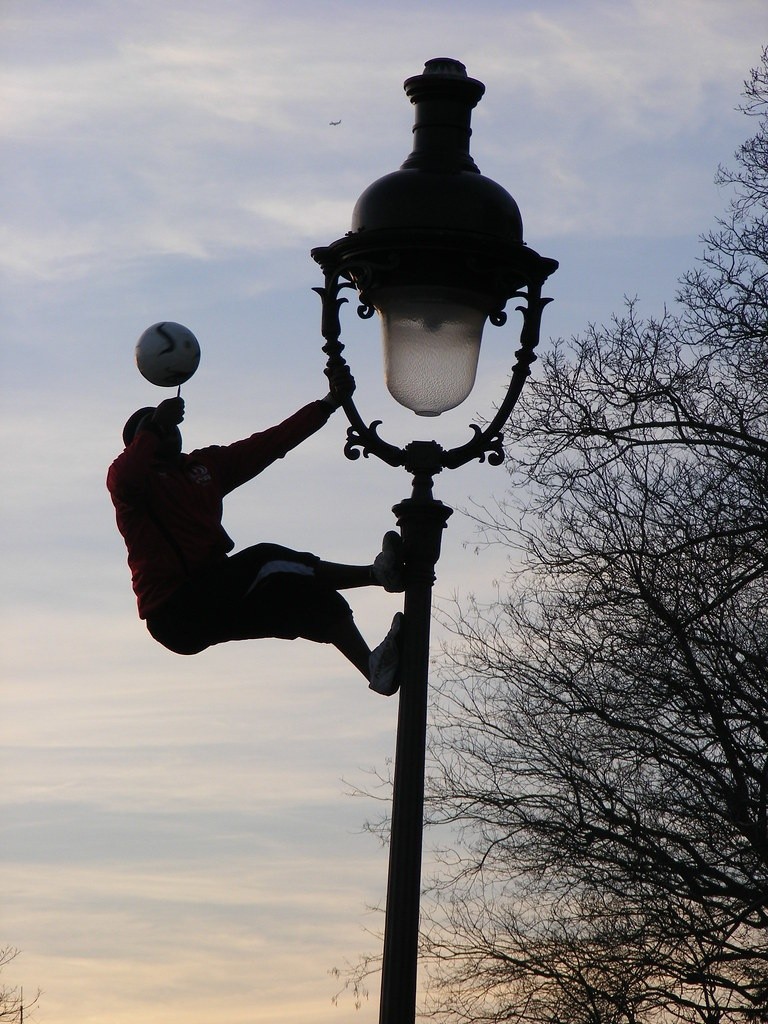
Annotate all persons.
[107,365,406,696]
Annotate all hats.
[124,407,157,447]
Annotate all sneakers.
[368,611,403,696]
[373,530,408,593]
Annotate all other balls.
[134,320,203,389]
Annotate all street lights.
[307,56,560,1023]
